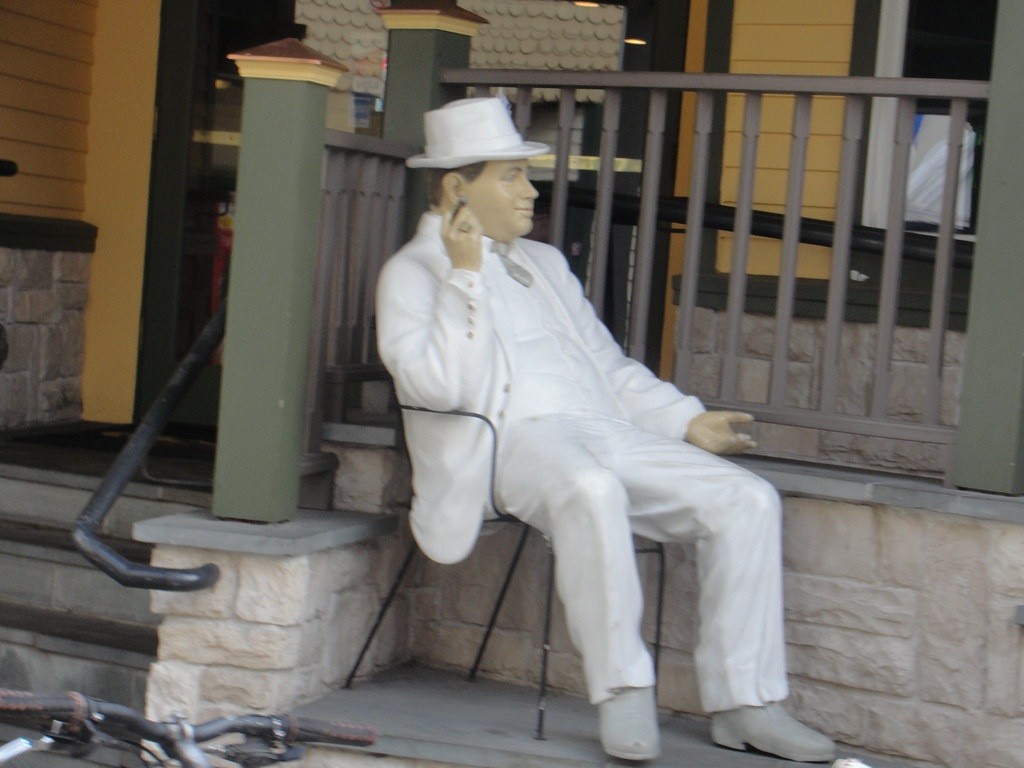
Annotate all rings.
[459,222,472,232]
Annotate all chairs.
[340,377,667,741]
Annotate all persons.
[375,99,837,762]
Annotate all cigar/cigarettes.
[451,197,466,220]
